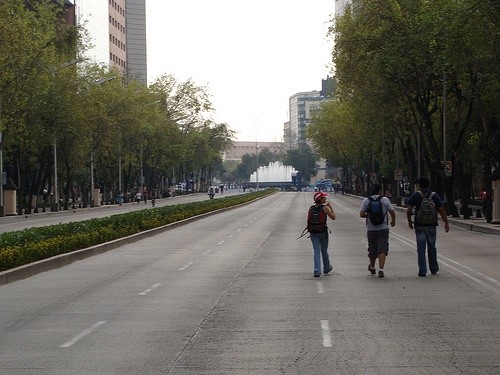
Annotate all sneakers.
[434,270,439,275]
[368,264,376,275]
[378,269,384,278]
[418,273,426,277]
[313,270,323,277]
[324,264,332,274]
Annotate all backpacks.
[367,194,383,226]
[417,191,437,223]
[307,205,327,233]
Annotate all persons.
[57,178,193,209]
[406,176,449,277]
[359,184,396,277]
[307,193,336,277]
[280,176,342,193]
[206,184,270,200]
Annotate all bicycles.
[207,193,215,200]
[150,196,156,207]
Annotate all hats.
[313,192,328,201]
